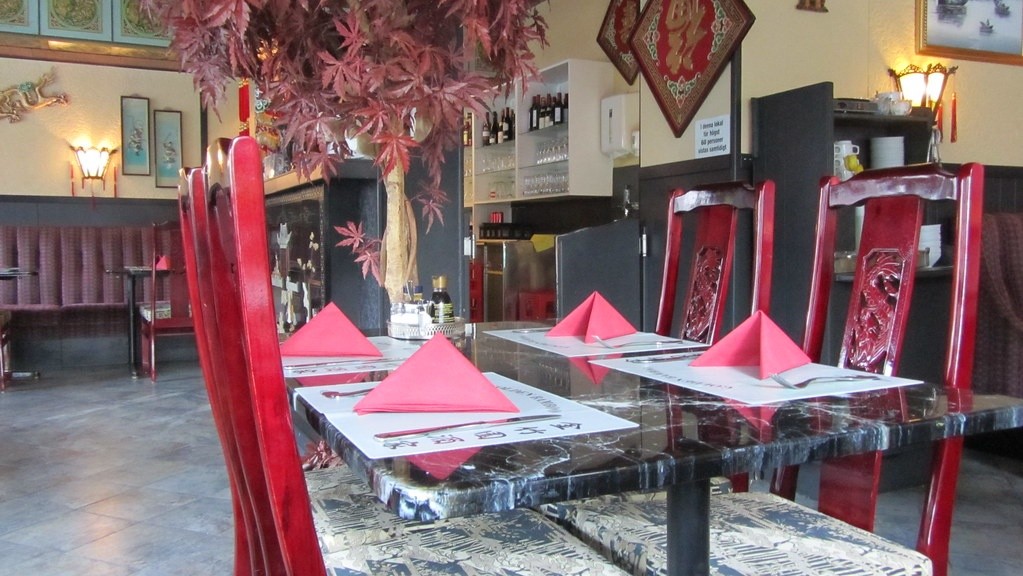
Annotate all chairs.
[176,166,366,576]
[533,160,986,576]
[203,137,634,576]
[537,179,777,523]
[139,220,200,382]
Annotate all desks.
[0,269,41,393]
[104,265,176,380]
[279,317,1023,576]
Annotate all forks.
[592,335,684,349]
[768,374,879,390]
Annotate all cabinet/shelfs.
[476,238,556,322]
[464,57,614,246]
[752,81,985,500]
[262,157,385,334]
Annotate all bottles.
[482,107,515,147]
[429,275,455,323]
[463,115,472,146]
[529,92,568,132]
[522,172,569,196]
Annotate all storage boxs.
[469,260,484,322]
[917,224,943,268]
[139,302,171,322]
[504,287,518,321]
[833,250,929,273]
[518,291,556,319]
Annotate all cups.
[534,136,568,165]
[833,139,864,183]
[481,151,515,172]
[487,180,516,199]
[869,136,904,169]
[871,91,912,116]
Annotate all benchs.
[0,215,194,329]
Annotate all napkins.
[687,308,812,381]
[723,398,778,431]
[403,447,484,482]
[294,371,368,387]
[568,354,623,385]
[277,300,384,358]
[543,289,638,344]
[352,331,520,416]
[156,254,172,269]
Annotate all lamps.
[886,62,959,144]
[69,145,118,212]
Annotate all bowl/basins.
[917,224,942,267]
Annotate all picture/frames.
[626,0,757,140]
[0,0,40,36]
[39,0,113,42]
[913,0,1023,66]
[596,0,643,85]
[112,0,173,47]
[120,95,151,176]
[154,109,184,188]
